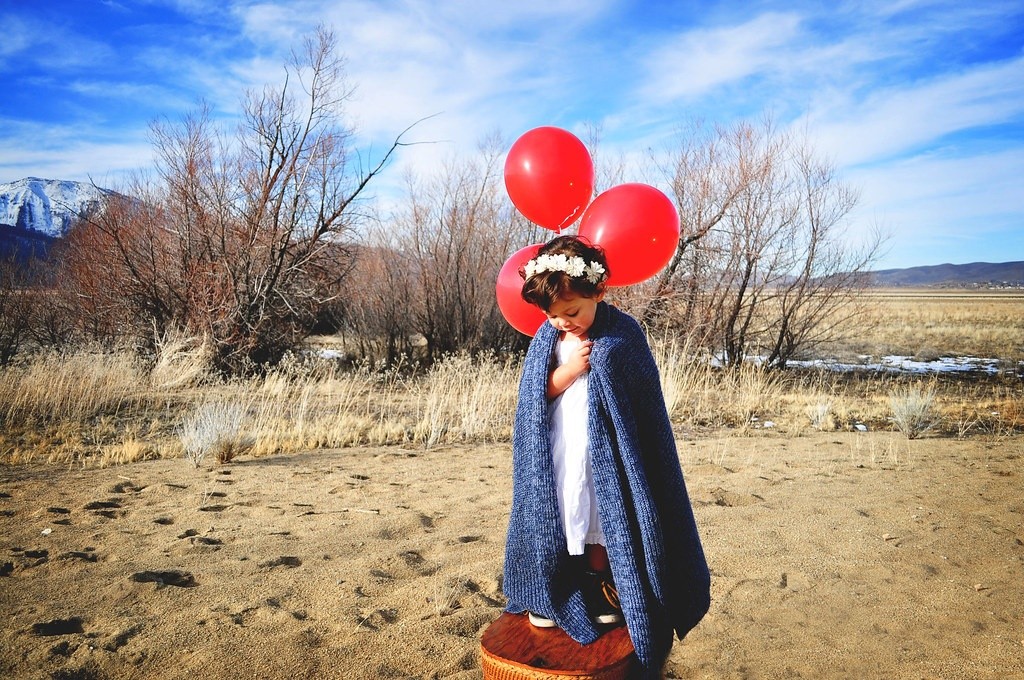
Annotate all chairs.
[480,614,639,680]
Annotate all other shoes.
[584,569,624,624]
[528,611,556,628]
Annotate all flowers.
[525,254,606,284]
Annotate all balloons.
[579,183,680,287]
[505,127,594,235]
[496,243,556,335]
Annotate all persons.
[504,235,711,680]
